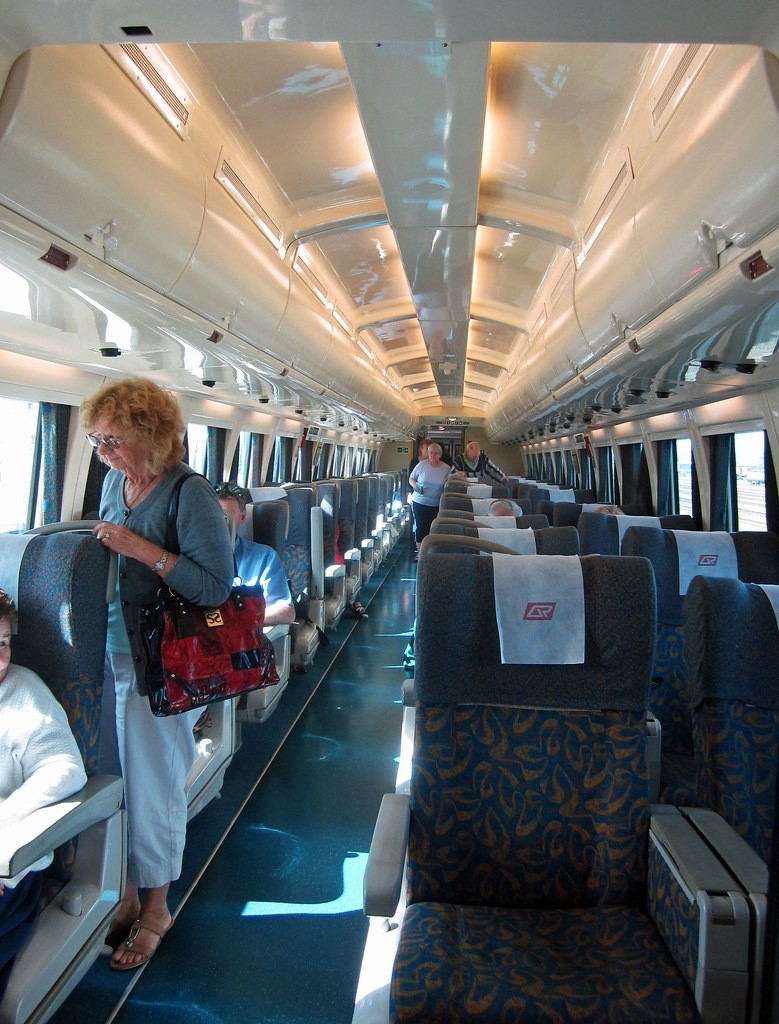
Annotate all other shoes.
[414,555,419,561]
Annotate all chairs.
[0,472,418,1024]
[358,477,779,1024]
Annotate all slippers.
[111,919,174,969]
[106,918,137,935]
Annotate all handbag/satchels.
[139,473,280,717]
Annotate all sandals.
[351,602,369,619]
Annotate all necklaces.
[128,476,156,507]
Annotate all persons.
[213,483,296,710]
[448,442,508,481]
[408,439,451,561]
[81,378,234,970]
[0,587,88,1009]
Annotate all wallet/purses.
[413,482,423,494]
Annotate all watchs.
[152,550,169,573]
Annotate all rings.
[104,532,110,538]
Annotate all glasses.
[216,484,245,500]
[85,430,129,449]
